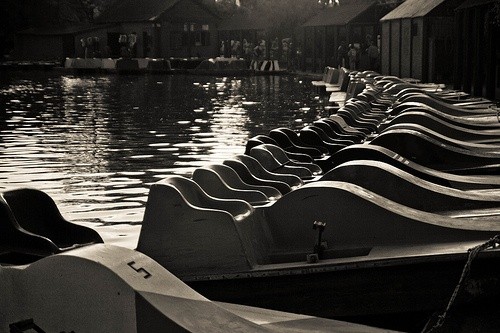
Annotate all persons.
[71,28,383,79]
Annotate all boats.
[0,61,500,332]
